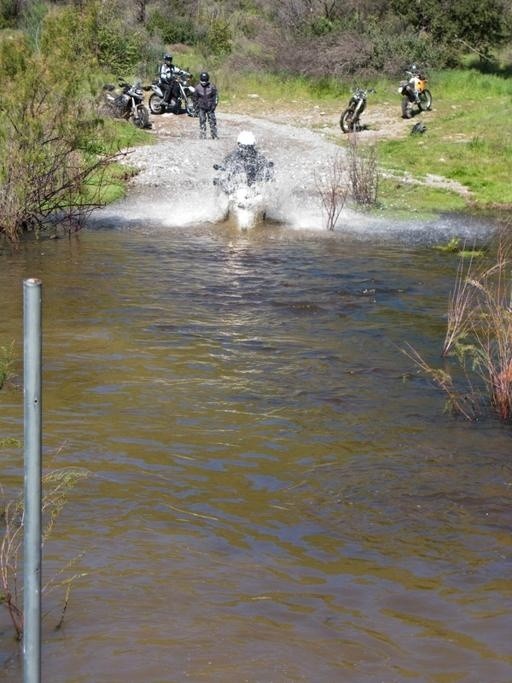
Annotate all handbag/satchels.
[411,123,427,136]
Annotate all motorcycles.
[147,71,199,115]
[98,76,153,133]
[395,65,434,120]
[334,85,377,134]
[213,161,278,227]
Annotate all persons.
[157,52,193,106]
[192,70,220,139]
[211,128,279,224]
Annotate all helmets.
[236,131,257,157]
[200,73,209,81]
[164,54,173,60]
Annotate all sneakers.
[200,133,219,141]
